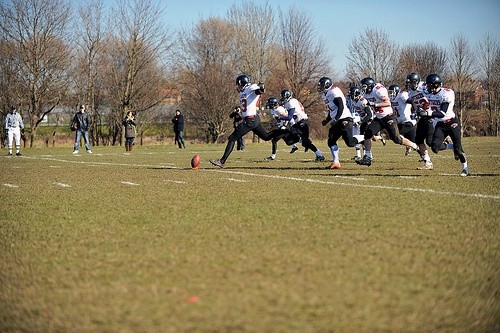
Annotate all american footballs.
[73,124,78,129]
[191,154,200,168]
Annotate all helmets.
[319,77,333,90]
[426,74,442,84]
[236,75,251,91]
[405,72,420,84]
[361,77,376,92]
[268,98,277,106]
[349,87,360,100]
[280,90,292,101]
[389,84,399,96]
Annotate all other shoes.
[209,159,224,168]
[86,150,92,153]
[305,135,469,177]
[16,153,21,156]
[290,147,298,153]
[7,154,12,155]
[72,150,78,154]
[268,156,276,160]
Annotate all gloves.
[410,113,416,119]
[331,120,336,126]
[322,120,327,126]
[260,85,265,90]
[275,116,279,120]
[367,101,375,107]
[420,109,433,117]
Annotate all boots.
[126,143,133,151]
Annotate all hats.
[11,105,16,109]
[80,105,85,109]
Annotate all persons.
[122,111,137,152]
[171,110,185,148]
[4,105,24,156]
[209,72,468,177]
[70,105,92,154]
